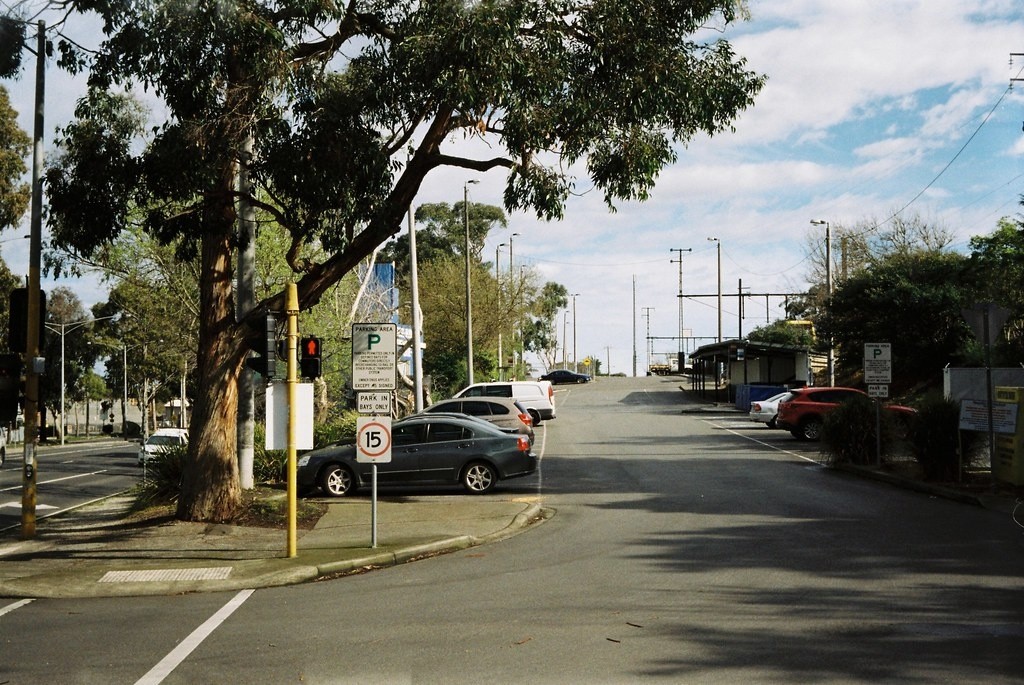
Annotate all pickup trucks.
[650,359,693,375]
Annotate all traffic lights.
[245,313,275,381]
[301,337,321,378]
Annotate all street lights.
[520,264,529,377]
[510,231,522,379]
[86,340,165,440]
[45,312,132,445]
[571,292,580,373]
[708,237,723,385]
[463,179,480,385]
[809,220,832,387]
[496,242,508,381]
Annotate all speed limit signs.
[356,423,391,456]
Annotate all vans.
[451,381,556,427]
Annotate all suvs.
[419,397,535,446]
[774,387,920,442]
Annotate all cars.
[139,427,188,466]
[542,370,590,384]
[749,391,794,429]
[295,412,538,497]
